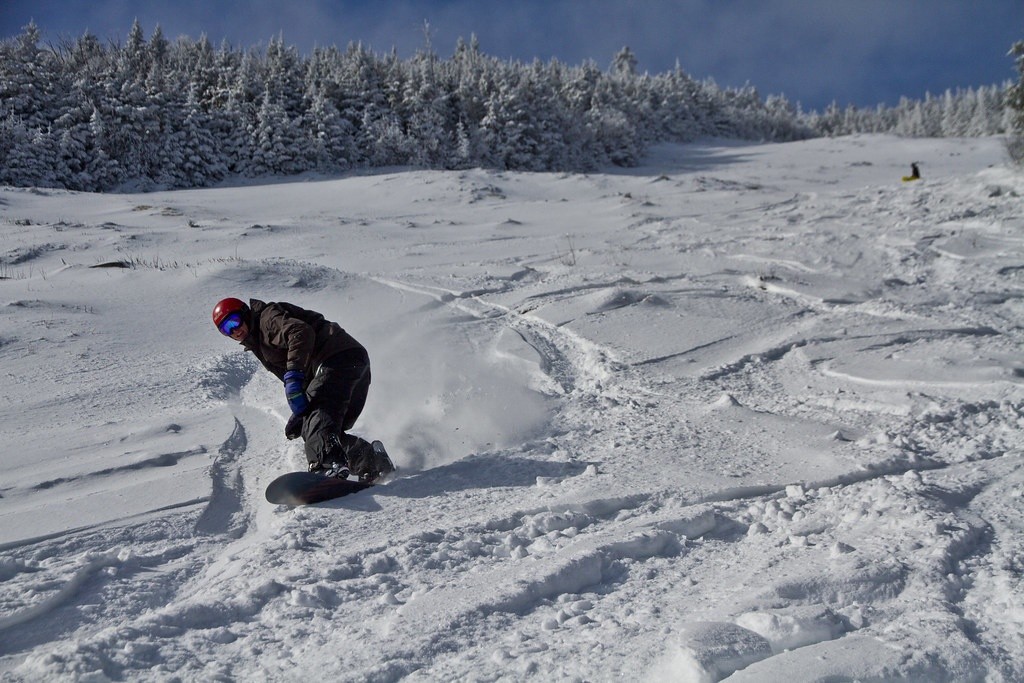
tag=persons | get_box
[902,162,920,181]
[212,297,395,483]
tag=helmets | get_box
[212,298,249,336]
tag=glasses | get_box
[219,311,244,335]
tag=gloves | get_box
[283,372,310,417]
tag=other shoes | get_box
[315,462,349,479]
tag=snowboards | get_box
[264,469,374,507]
